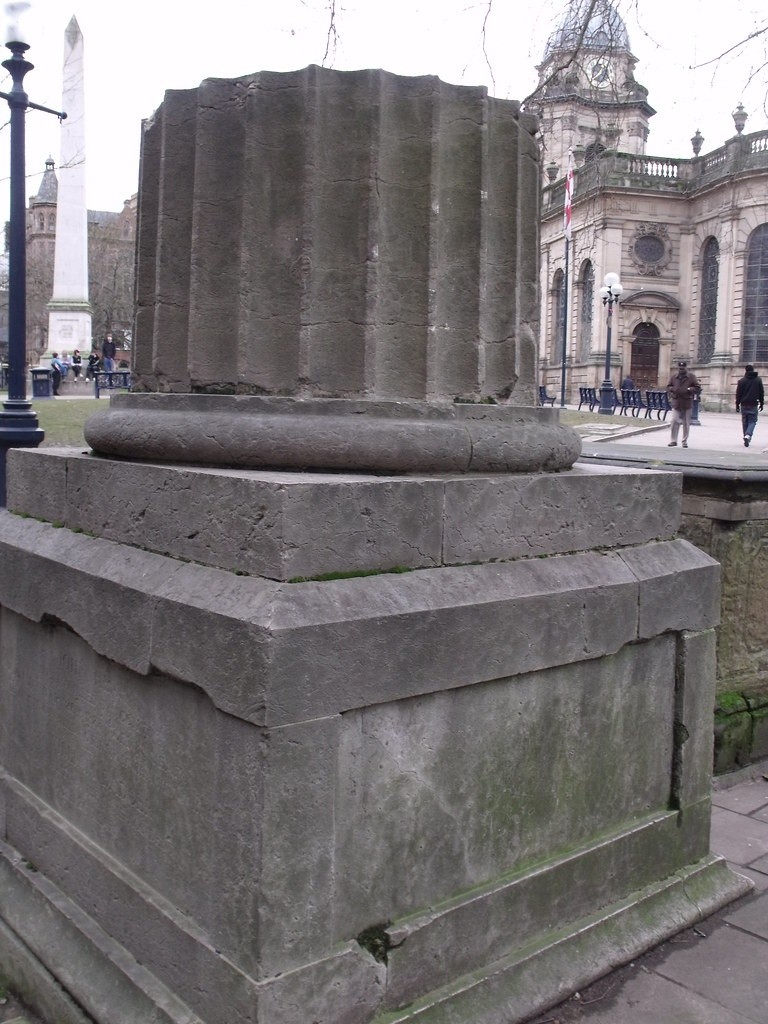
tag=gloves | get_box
[736,406,740,412]
[758,405,763,412]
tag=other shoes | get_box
[80,375,83,377]
[86,378,89,383]
[93,378,95,381]
[53,392,60,395]
[683,444,688,448]
[74,379,77,381]
[668,442,677,446]
[743,435,750,447]
[62,377,66,383]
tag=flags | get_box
[564,161,575,237]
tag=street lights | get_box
[596,272,622,414]
[0,2,67,505]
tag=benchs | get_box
[539,386,556,407]
[94,371,131,398]
[620,389,651,419]
[611,389,622,414]
[645,390,672,421]
[578,388,600,412]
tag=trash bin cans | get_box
[29,369,55,396]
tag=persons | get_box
[61,351,70,379]
[102,333,116,381]
[621,375,634,405]
[737,365,764,447]
[71,349,83,382]
[668,362,700,449]
[51,352,63,396]
[86,352,100,383]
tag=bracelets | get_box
[688,388,690,391]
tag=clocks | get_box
[586,56,615,88]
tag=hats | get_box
[678,362,687,368]
